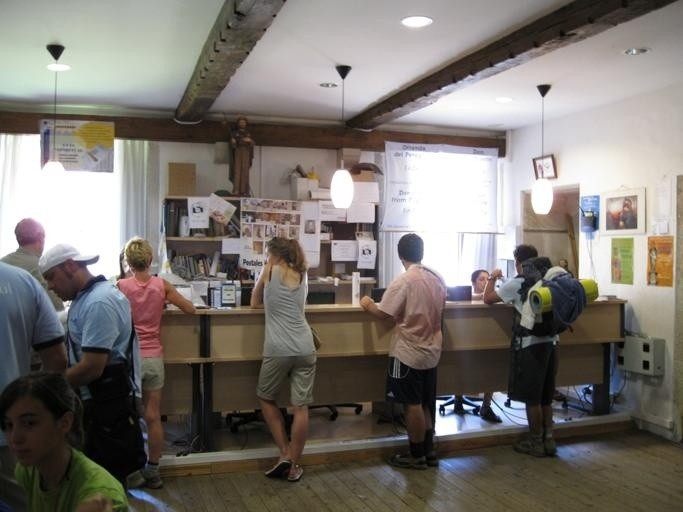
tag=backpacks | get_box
[513,256,569,338]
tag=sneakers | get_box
[146,471,163,489]
[514,437,544,457]
[542,437,558,456]
[425,450,438,468]
[387,451,428,472]
[125,471,144,488]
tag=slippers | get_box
[265,460,291,478]
[287,464,304,482]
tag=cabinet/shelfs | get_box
[159,195,380,303]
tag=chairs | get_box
[305,291,363,422]
[436,286,484,416]
[505,388,568,409]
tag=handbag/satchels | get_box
[66,321,148,481]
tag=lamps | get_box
[37,44,71,214]
[531,84,553,215]
[330,65,354,209]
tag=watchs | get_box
[487,274,496,281]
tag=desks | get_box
[157,306,206,445]
[201,300,443,446]
[433,296,628,433]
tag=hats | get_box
[13,217,44,246]
[39,241,100,273]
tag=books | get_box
[163,202,240,237]
[165,253,239,279]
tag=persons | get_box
[250,236,318,482]
[1,371,133,511]
[0,218,67,318]
[1,257,71,512]
[108,250,133,291]
[229,117,255,196]
[37,243,142,495]
[361,233,447,470]
[484,244,558,457]
[115,236,197,488]
[460,270,503,424]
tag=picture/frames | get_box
[532,154,557,181]
[599,187,646,236]
